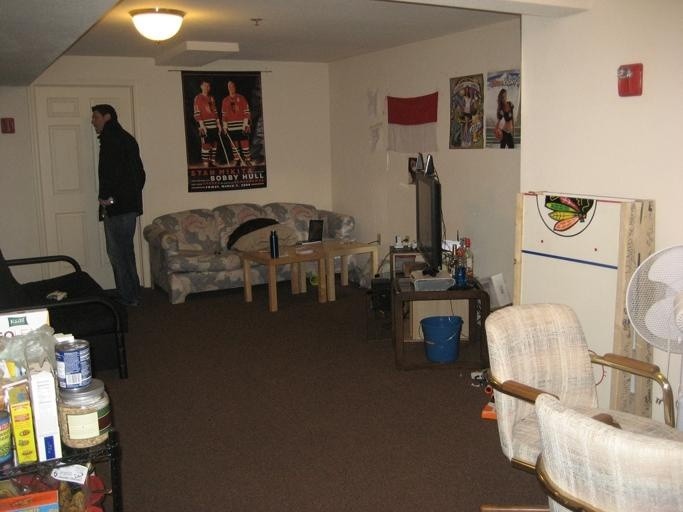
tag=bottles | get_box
[270,229,280,259]
[58,378,112,449]
[450,236,474,288]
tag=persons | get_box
[459,85,476,147]
[495,89,514,149]
[194,78,220,168]
[221,80,255,167]
[92,104,145,307]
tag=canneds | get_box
[54,339,92,392]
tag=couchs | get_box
[143,202,356,305]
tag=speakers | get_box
[423,153,436,177]
[414,151,424,171]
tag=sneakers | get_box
[113,295,143,306]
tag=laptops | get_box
[297,219,323,245]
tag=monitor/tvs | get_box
[414,172,442,277]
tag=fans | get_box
[624,244,683,429]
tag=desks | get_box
[239,246,326,312]
[301,241,379,304]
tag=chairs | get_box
[481,301,675,512]
[1,246,131,382]
[532,394,683,512]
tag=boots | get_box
[208,160,216,167]
[234,159,252,166]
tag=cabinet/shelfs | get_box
[0,428,124,512]
[392,269,491,370]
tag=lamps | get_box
[127,7,186,42]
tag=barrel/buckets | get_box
[419,315,464,364]
